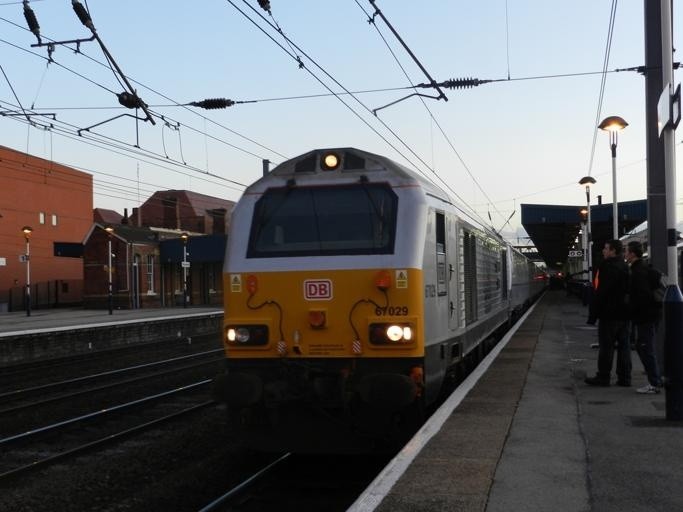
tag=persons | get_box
[589,248,619,352]
[548,270,594,307]
[625,240,664,394]
[583,238,633,387]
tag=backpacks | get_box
[648,267,669,312]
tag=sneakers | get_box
[590,341,600,349]
[584,376,662,394]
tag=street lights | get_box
[180,233,189,308]
[598,117,629,240]
[579,177,596,307]
[104,225,114,315]
[21,226,33,317]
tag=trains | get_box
[223,148,546,407]
